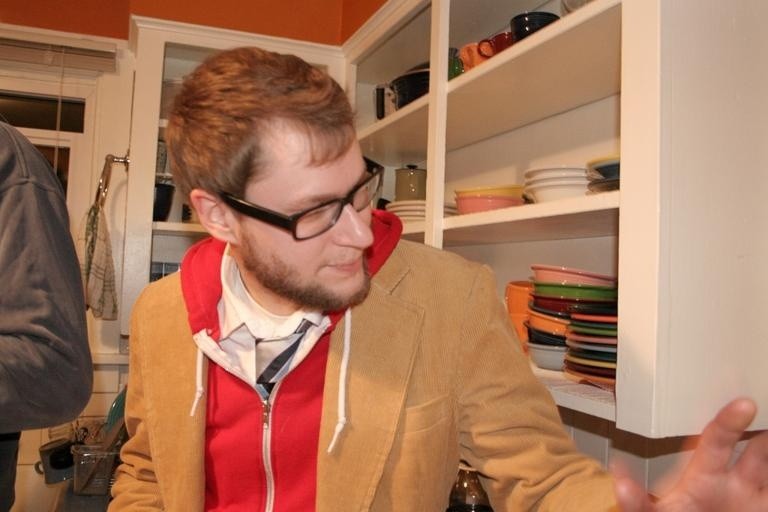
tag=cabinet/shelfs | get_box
[121,13,344,338]
[342,0,767,438]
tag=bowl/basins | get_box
[503,280,533,354]
[523,263,616,372]
[521,166,591,204]
[389,60,430,110]
[455,185,526,216]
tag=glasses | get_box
[208,155,384,242]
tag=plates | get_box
[107,385,126,428]
[563,311,617,394]
[386,199,457,227]
[589,161,620,191]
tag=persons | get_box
[107,45,768,512]
[0,122,93,512]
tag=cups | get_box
[460,31,513,73]
[48,423,77,447]
[155,181,175,222]
[508,11,560,43]
[34,437,75,486]
